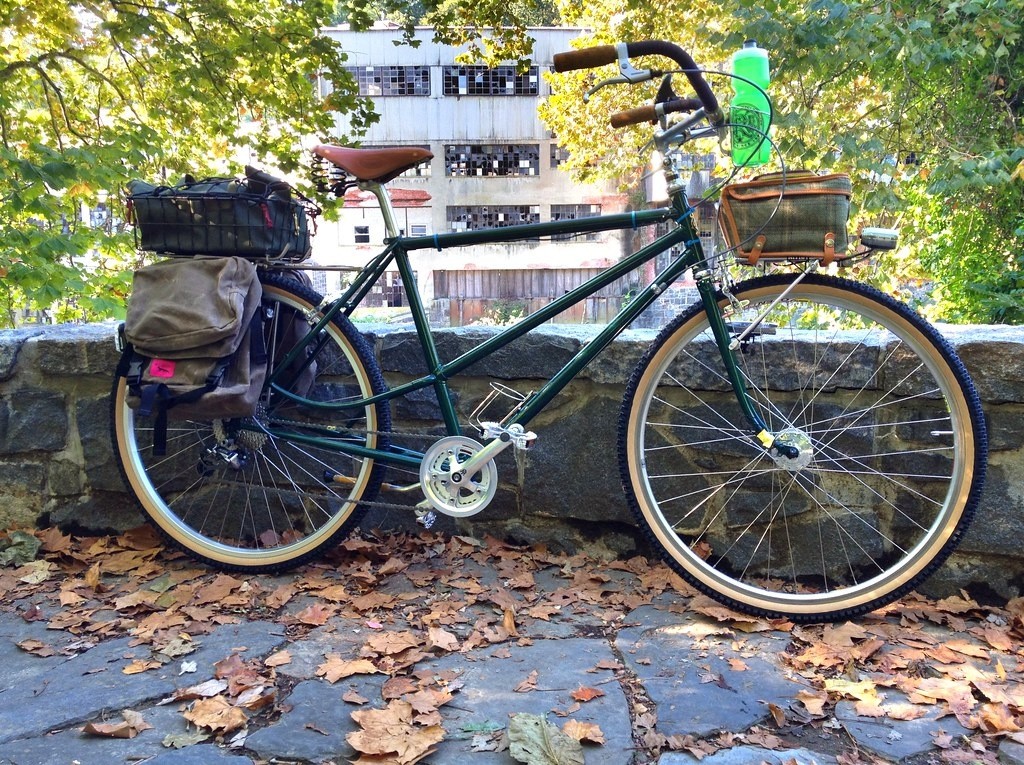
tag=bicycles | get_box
[108,40,988,624]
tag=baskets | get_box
[126,196,310,261]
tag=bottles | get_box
[731,39,770,167]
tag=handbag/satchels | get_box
[123,255,263,358]
[125,321,267,419]
[718,169,851,265]
[264,267,318,408]
